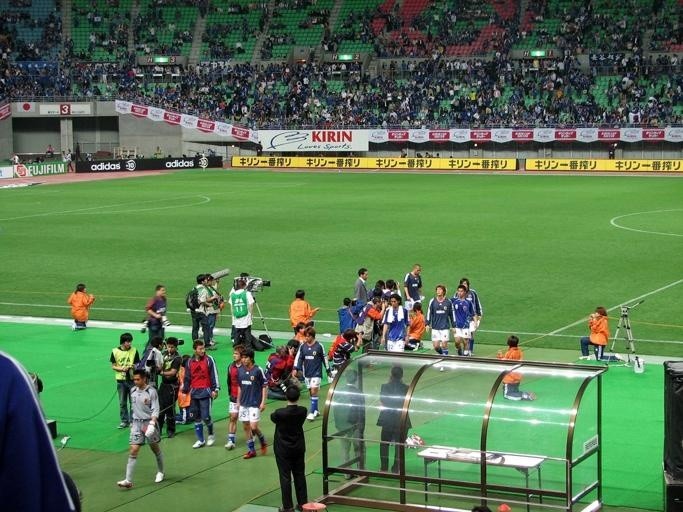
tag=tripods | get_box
[606,306,641,368]
[252,293,269,334]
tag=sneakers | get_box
[210,341,215,346]
[225,441,235,448]
[344,473,354,479]
[579,356,590,360]
[413,343,419,351]
[419,343,423,349]
[314,411,320,416]
[193,439,205,448]
[296,504,303,511]
[205,346,217,350]
[71,320,75,331]
[207,435,214,445]
[528,391,536,400]
[614,353,625,362]
[168,431,174,438]
[440,366,446,371]
[243,450,256,459]
[279,507,295,512]
[117,422,129,429]
[261,441,268,454]
[155,472,165,482]
[117,480,132,488]
[306,413,314,421]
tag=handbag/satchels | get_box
[240,332,265,351]
[259,335,272,347]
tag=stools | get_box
[301,502,329,512]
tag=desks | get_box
[417,445,547,512]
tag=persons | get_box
[1,1,682,172]
[68,283,95,330]
[497,336,536,401]
[0,355,81,512]
[108,264,482,509]
[578,307,623,361]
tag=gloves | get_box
[145,420,156,437]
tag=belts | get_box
[134,419,151,422]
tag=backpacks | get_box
[186,287,200,309]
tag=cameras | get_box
[354,330,364,341]
[208,268,229,281]
[246,277,270,292]
[164,338,184,346]
